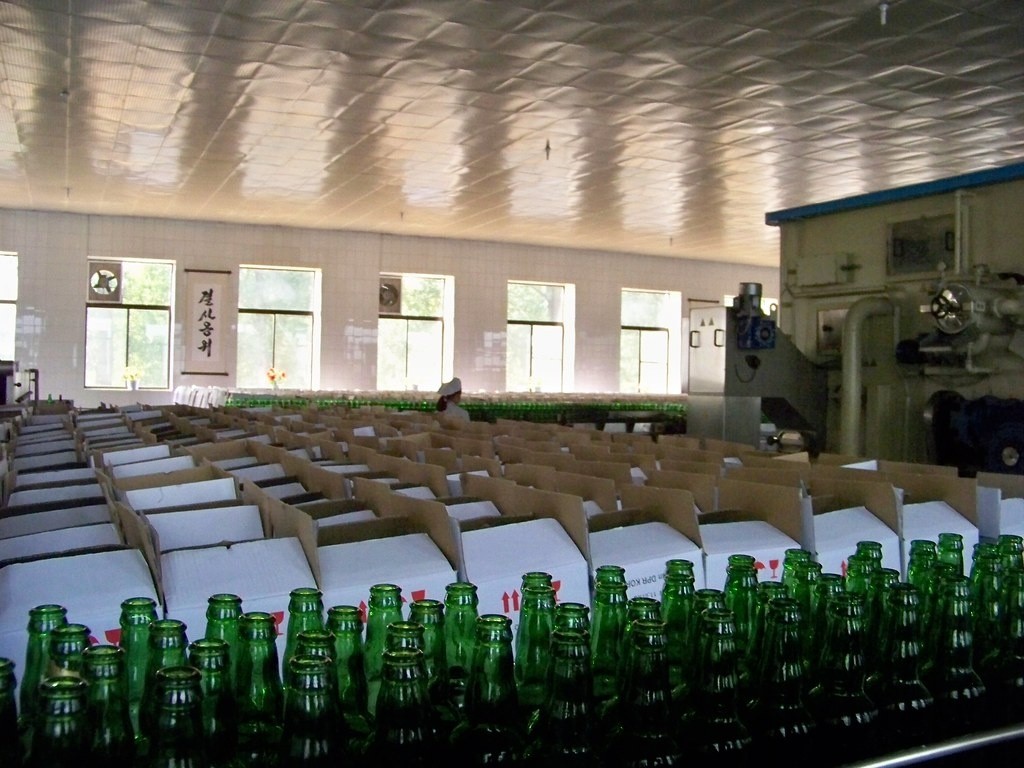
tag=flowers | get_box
[122,366,141,379]
[266,368,285,383]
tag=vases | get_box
[274,381,279,389]
[129,379,139,389]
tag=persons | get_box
[436,377,470,423]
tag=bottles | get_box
[222,389,690,416]
[47,394,52,407]
[0,528,1024,766]
[100,402,113,409]
[59,394,63,402]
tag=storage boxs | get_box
[0,399,1024,717]
